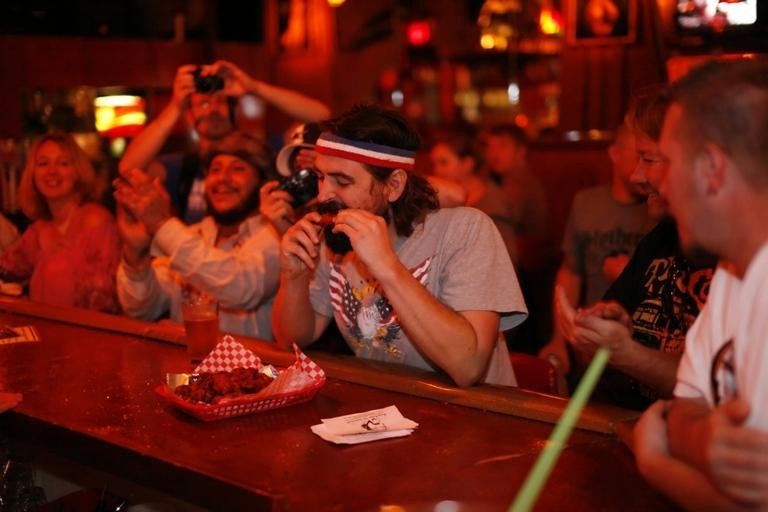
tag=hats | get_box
[208,132,274,181]
[276,122,320,177]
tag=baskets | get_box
[154,367,327,422]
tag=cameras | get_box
[278,167,318,208]
[189,63,224,93]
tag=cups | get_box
[181,284,221,362]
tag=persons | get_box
[630,59,767,512]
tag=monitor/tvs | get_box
[675,0,768,37]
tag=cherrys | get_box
[174,367,274,403]
